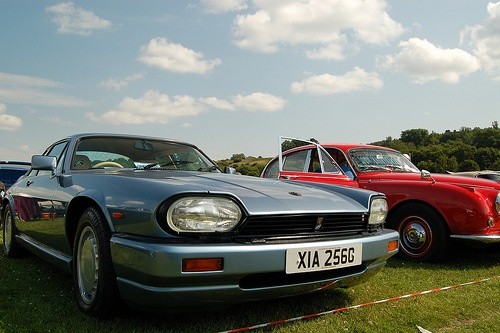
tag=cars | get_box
[1,132,398,317]
[261,136,499,261]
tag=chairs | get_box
[71,155,91,170]
